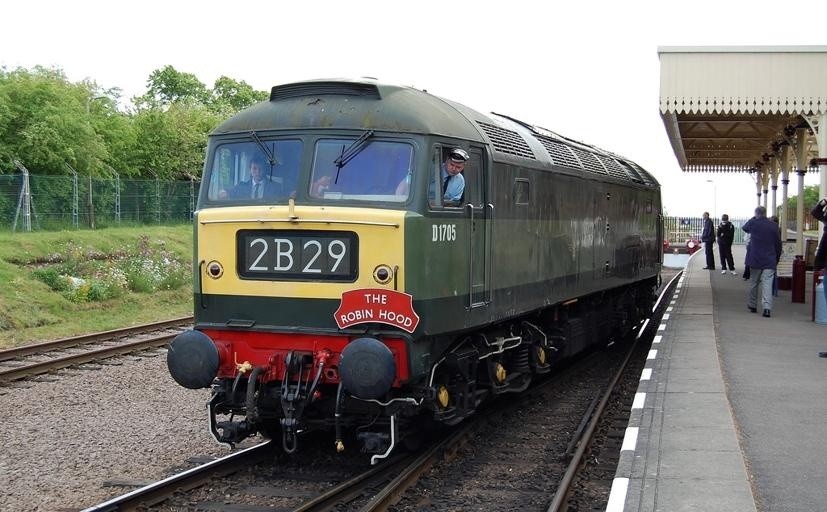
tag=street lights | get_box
[85,90,116,232]
[705,178,716,243]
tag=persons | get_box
[811,198,827,358]
[717,214,737,275]
[742,206,782,318]
[743,232,751,281]
[701,212,716,269]
[219,158,283,200]
[395,147,469,201]
[770,215,779,296]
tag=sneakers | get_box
[762,309,771,317]
[720,270,726,274]
[750,309,757,313]
[730,270,737,275]
[702,265,715,270]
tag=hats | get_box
[448,148,470,163]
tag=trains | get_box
[164,74,664,464]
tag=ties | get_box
[253,183,259,199]
[443,176,450,194]
